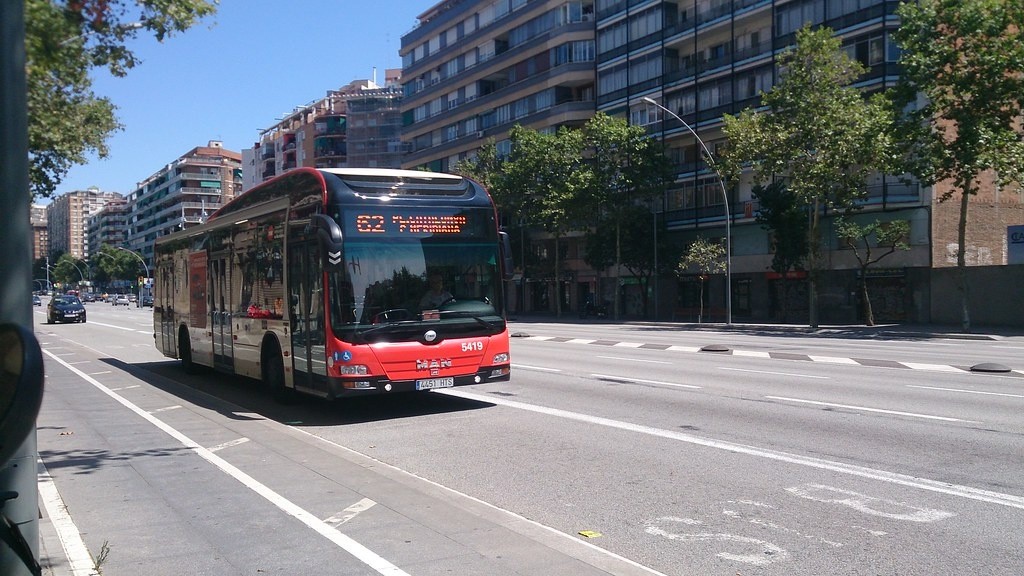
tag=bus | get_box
[154,167,510,406]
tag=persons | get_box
[419,275,453,309]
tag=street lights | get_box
[63,259,84,281]
[637,95,731,324]
[98,252,115,263]
[118,246,151,306]
[41,266,57,282]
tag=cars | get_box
[135,295,154,308]
[126,293,137,302]
[33,289,64,297]
[32,295,41,306]
[112,295,130,307]
[66,290,117,303]
[47,295,86,324]
